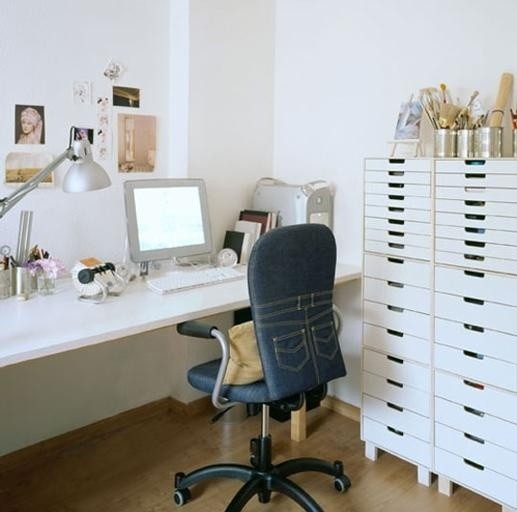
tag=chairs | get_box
[173,222,351,511]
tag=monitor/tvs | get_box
[124,178,213,273]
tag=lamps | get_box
[0,140,112,218]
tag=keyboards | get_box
[146,265,246,294]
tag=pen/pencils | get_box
[0,244,49,270]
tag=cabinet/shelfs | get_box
[361,157,517,512]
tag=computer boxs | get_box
[251,177,333,229]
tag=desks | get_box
[0,248,363,370]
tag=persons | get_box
[75,128,89,145]
[16,106,43,144]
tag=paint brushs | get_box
[416,84,488,130]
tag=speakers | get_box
[218,248,238,268]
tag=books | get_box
[221,207,278,265]
